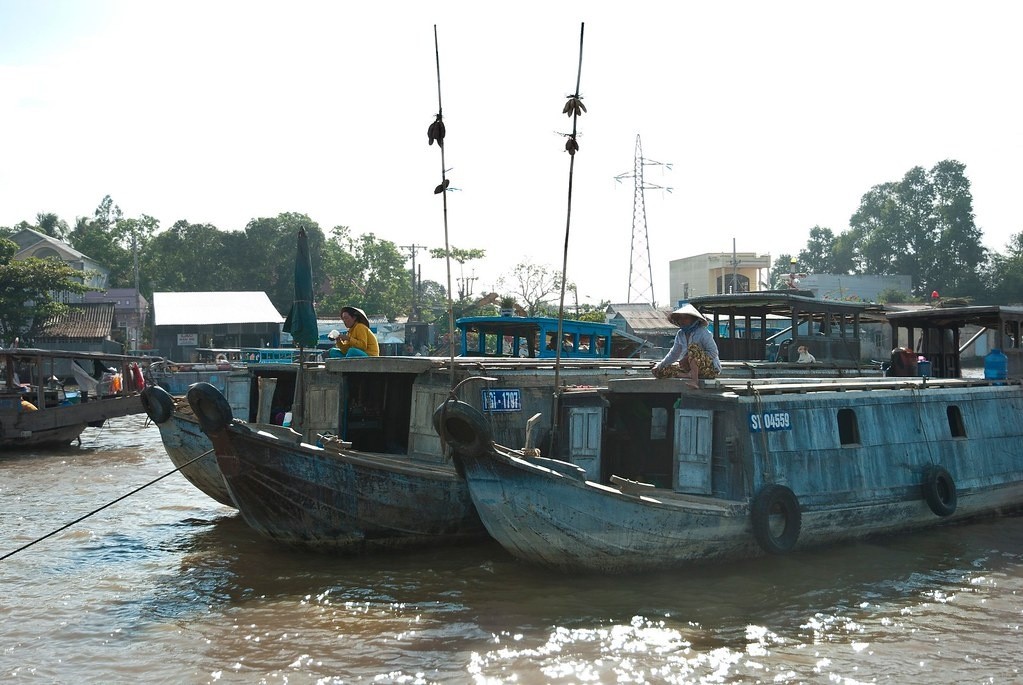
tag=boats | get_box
[168,348,248,371]
[433,21,1023,571]
[227,347,325,377]
[139,224,613,510]
[0,347,176,450]
[185,24,930,551]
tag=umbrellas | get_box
[282,226,318,421]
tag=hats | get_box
[48,375,59,383]
[341,306,369,328]
[668,304,708,328]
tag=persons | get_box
[420,343,429,355]
[769,341,778,361]
[406,344,414,355]
[652,305,722,390]
[329,306,379,358]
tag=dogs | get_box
[796,345,823,364]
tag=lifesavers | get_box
[750,484,802,555]
[140,386,175,423]
[186,382,233,431]
[432,399,492,457]
[216,353,227,364]
[923,465,957,517]
